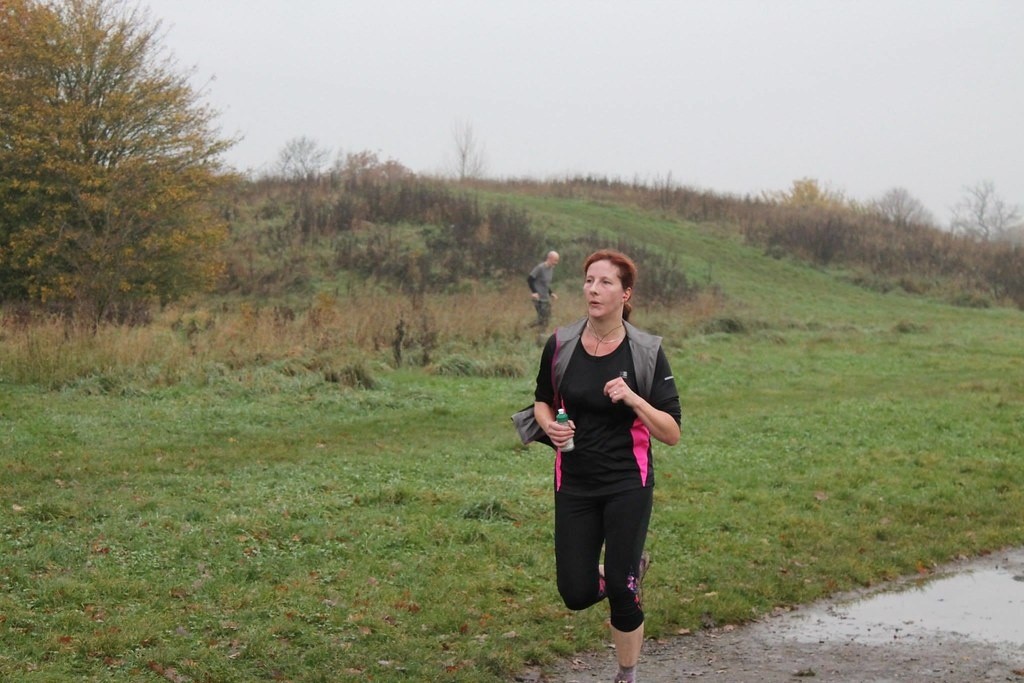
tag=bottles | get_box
[556,408,575,452]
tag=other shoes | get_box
[638,550,651,582]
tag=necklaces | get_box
[587,324,622,344]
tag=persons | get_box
[527,250,560,334]
[535,250,682,683]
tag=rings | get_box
[613,391,618,395]
